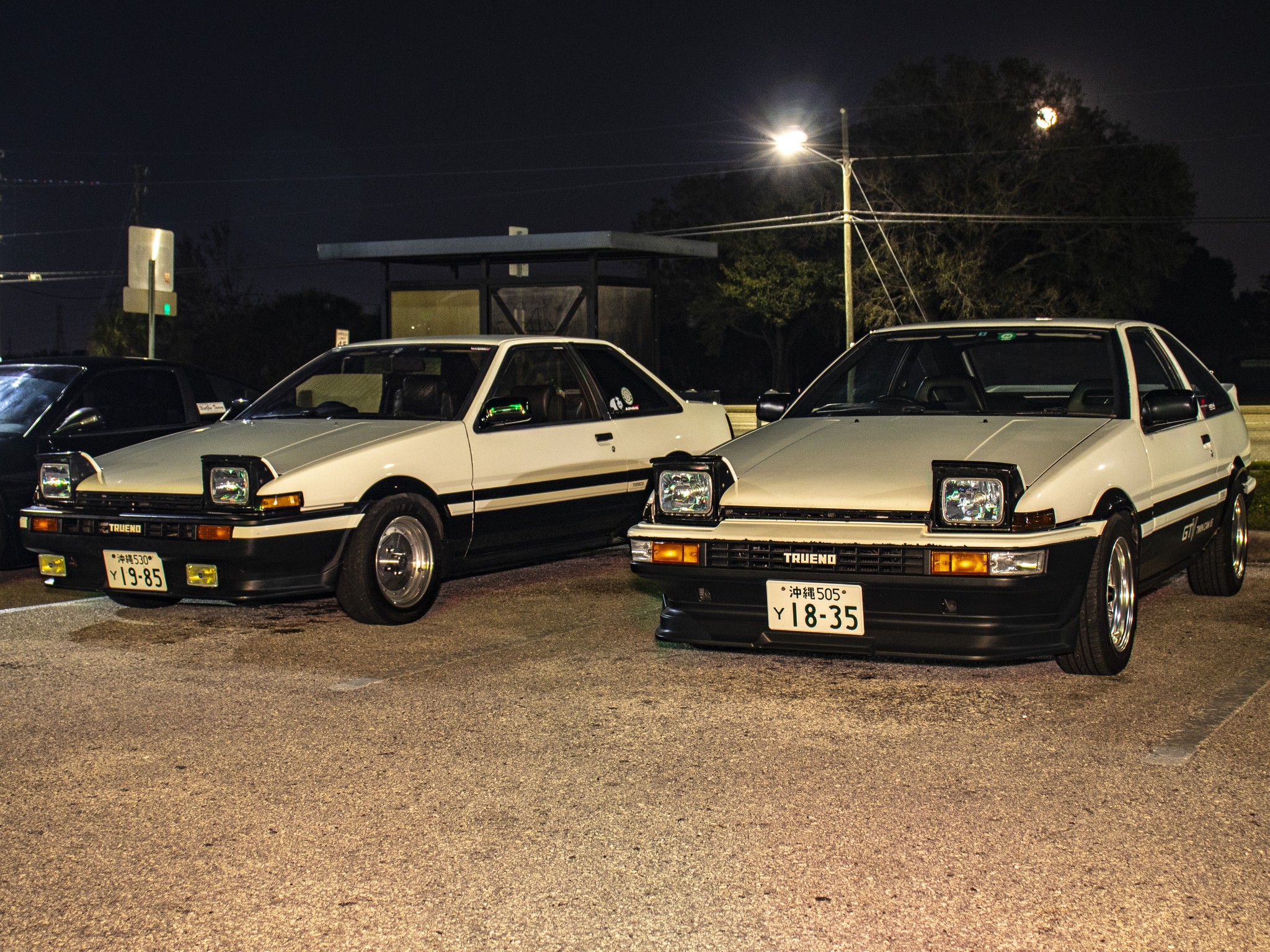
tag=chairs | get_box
[392,374,454,421]
[918,374,987,411]
[499,382,565,422]
[1063,377,1114,413]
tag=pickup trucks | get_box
[0,355,306,572]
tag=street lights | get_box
[774,108,861,406]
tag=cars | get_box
[627,318,1258,676]
[18,338,729,627]
